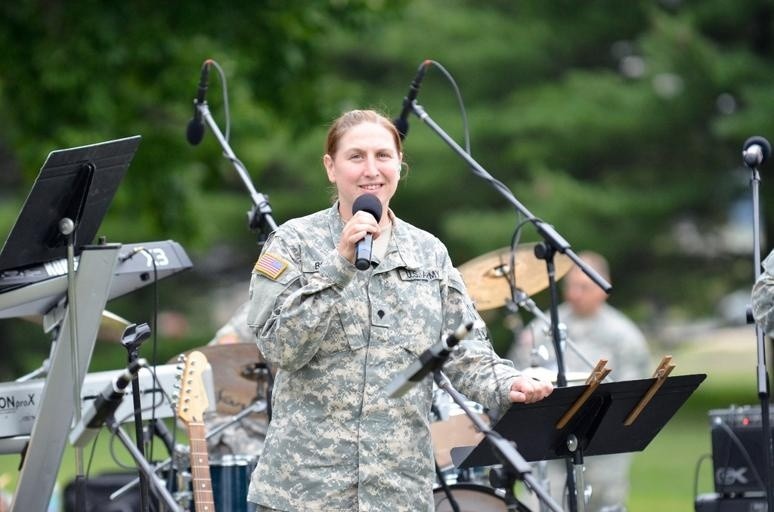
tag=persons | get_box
[502,251,655,512]
[247,109,554,512]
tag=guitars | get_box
[172,350,215,512]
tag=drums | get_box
[182,453,258,512]
[433,465,542,512]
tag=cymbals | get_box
[456,243,573,312]
[167,344,278,420]
[431,414,490,471]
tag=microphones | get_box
[386,326,467,399]
[393,66,422,142]
[741,135,771,168]
[352,194,382,270]
[69,359,141,448]
[187,67,209,145]
